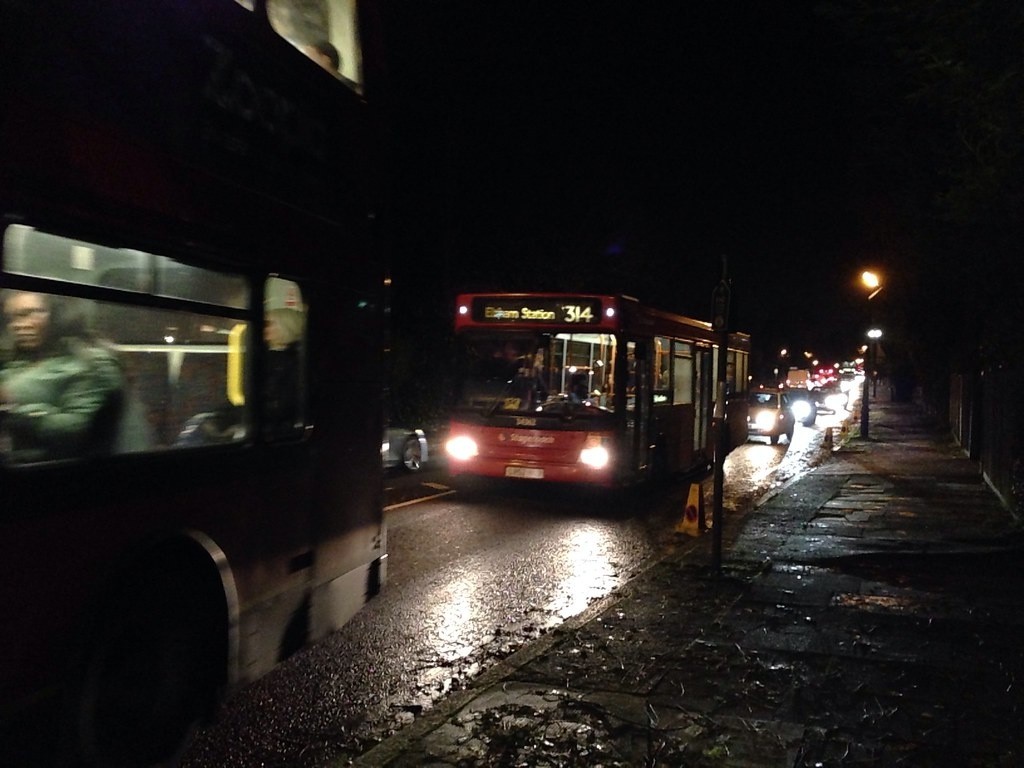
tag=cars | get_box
[785,388,820,429]
[806,367,859,412]
[378,414,431,474]
[750,388,795,445]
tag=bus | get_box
[442,288,751,494]
[0,215,399,768]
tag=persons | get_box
[1,287,129,465]
[264,321,287,353]
[726,376,735,396]
[658,364,670,388]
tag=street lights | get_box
[858,270,882,438]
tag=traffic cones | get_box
[672,483,709,539]
